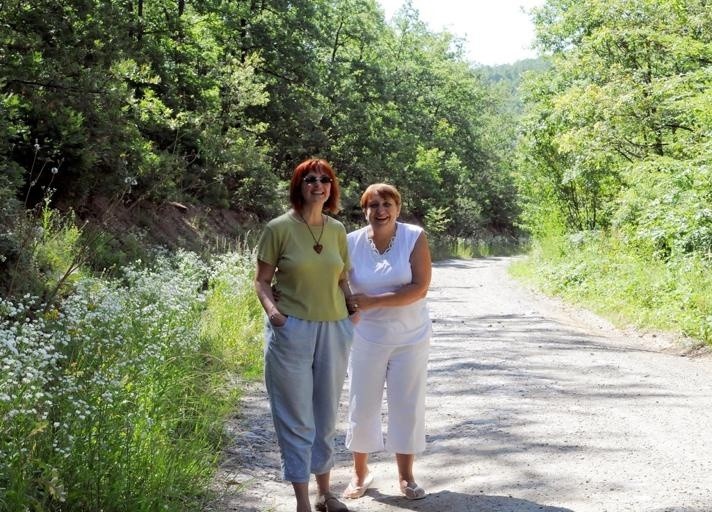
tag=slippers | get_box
[399,480,425,500]
[343,476,374,499]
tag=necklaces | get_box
[299,212,324,254]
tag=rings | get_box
[355,304,357,307]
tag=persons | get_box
[345,185,432,500]
[257,159,359,511]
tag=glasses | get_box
[303,175,333,183]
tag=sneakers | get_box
[315,496,347,512]
